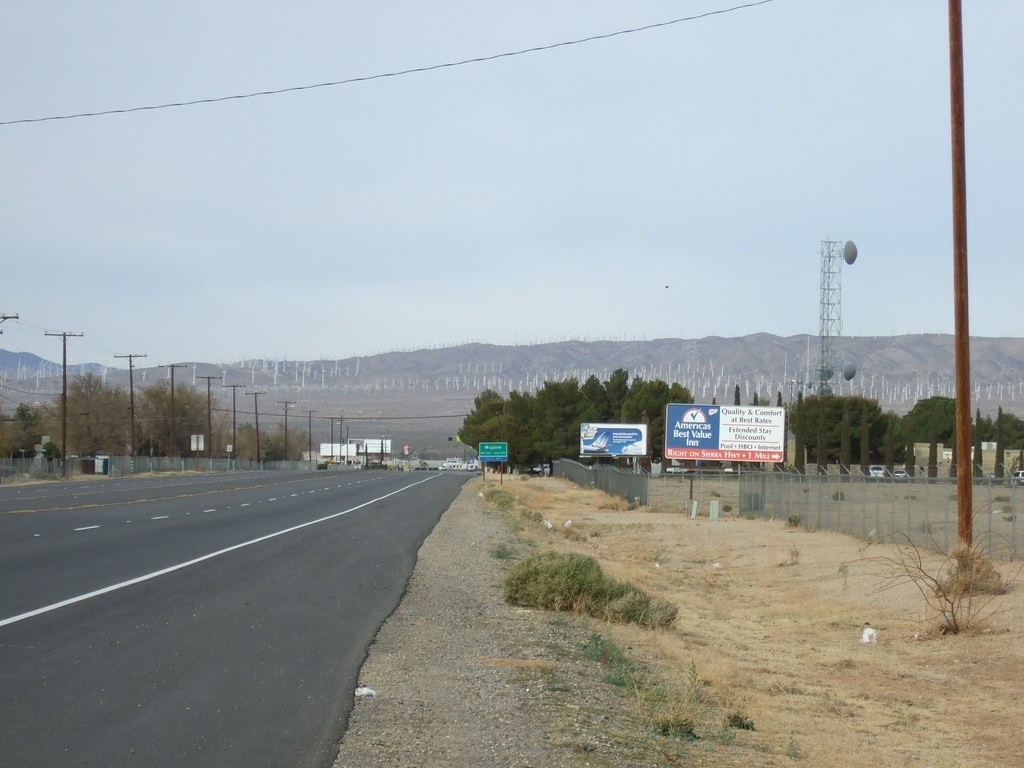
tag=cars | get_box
[892,470,906,478]
[1013,471,1024,483]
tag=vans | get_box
[869,464,885,478]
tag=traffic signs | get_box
[665,403,786,463]
